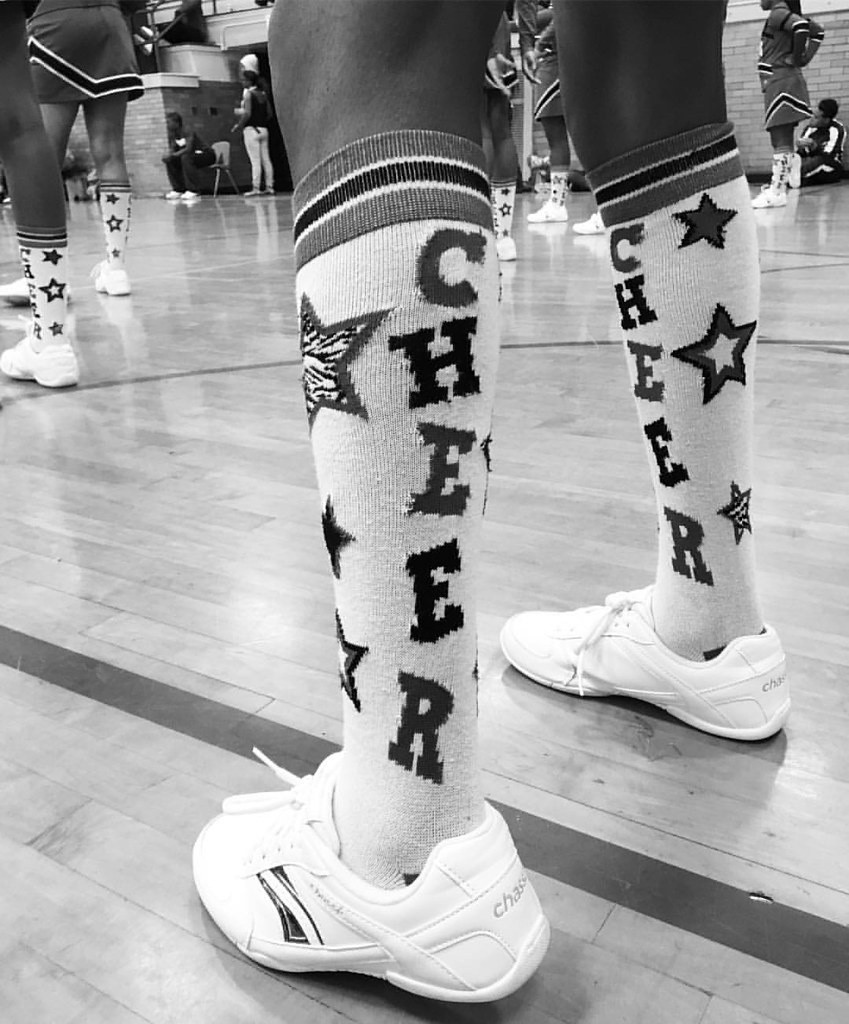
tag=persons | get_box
[192,0,791,1003]
[0,0,146,306]
[751,0,824,209]
[134,0,207,55]
[231,54,275,196]
[161,112,216,199]
[760,99,847,190]
[0,0,79,388]
[483,0,605,261]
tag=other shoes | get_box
[133,25,160,55]
[164,190,200,199]
[244,186,274,196]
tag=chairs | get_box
[208,141,240,199]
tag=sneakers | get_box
[496,235,516,260]
[0,321,80,389]
[527,199,568,223]
[753,188,787,208]
[497,579,795,742]
[95,269,132,296]
[0,277,31,306]
[572,212,606,235]
[789,153,801,188]
[188,749,553,1004]
